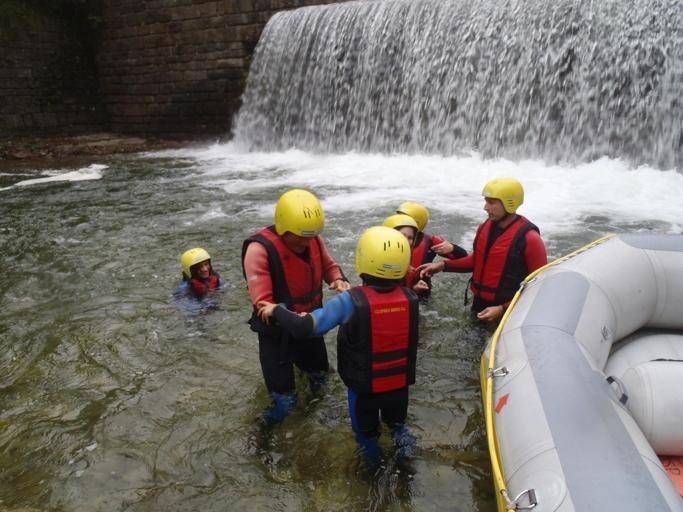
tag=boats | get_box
[478,231,681,511]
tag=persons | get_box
[414,177,549,332]
[171,248,222,311]
[241,189,350,425]
[251,227,419,456]
[395,201,467,294]
[382,214,429,293]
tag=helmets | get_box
[482,176,525,214]
[383,202,429,247]
[274,189,324,238]
[356,225,411,283]
[181,247,211,279]
